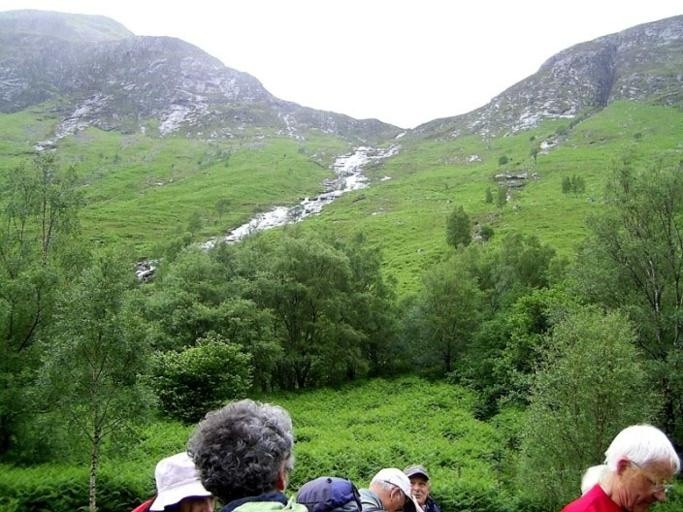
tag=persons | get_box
[295,468,411,512]
[144,450,218,512]
[185,399,310,512]
[556,423,680,512]
[401,465,439,512]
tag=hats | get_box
[149,453,213,511]
[297,464,429,512]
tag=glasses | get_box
[631,462,673,492]
